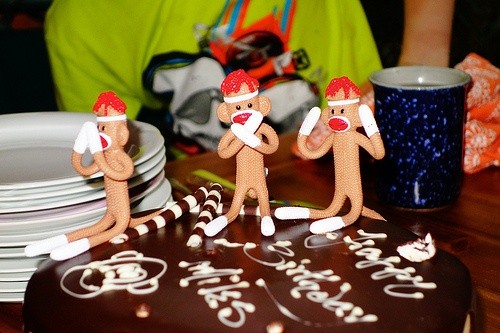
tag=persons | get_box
[45,0,381,162]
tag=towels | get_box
[453,52,500,174]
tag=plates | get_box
[0,112,173,303]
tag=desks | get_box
[0,130,500,333]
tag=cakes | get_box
[23,69,476,333]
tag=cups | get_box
[368,65,471,212]
